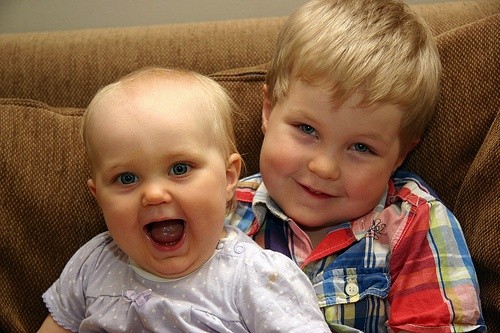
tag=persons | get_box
[224,1,487,333]
[37,66,330,333]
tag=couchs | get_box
[0,0,500,333]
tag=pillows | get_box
[0,14,500,333]
